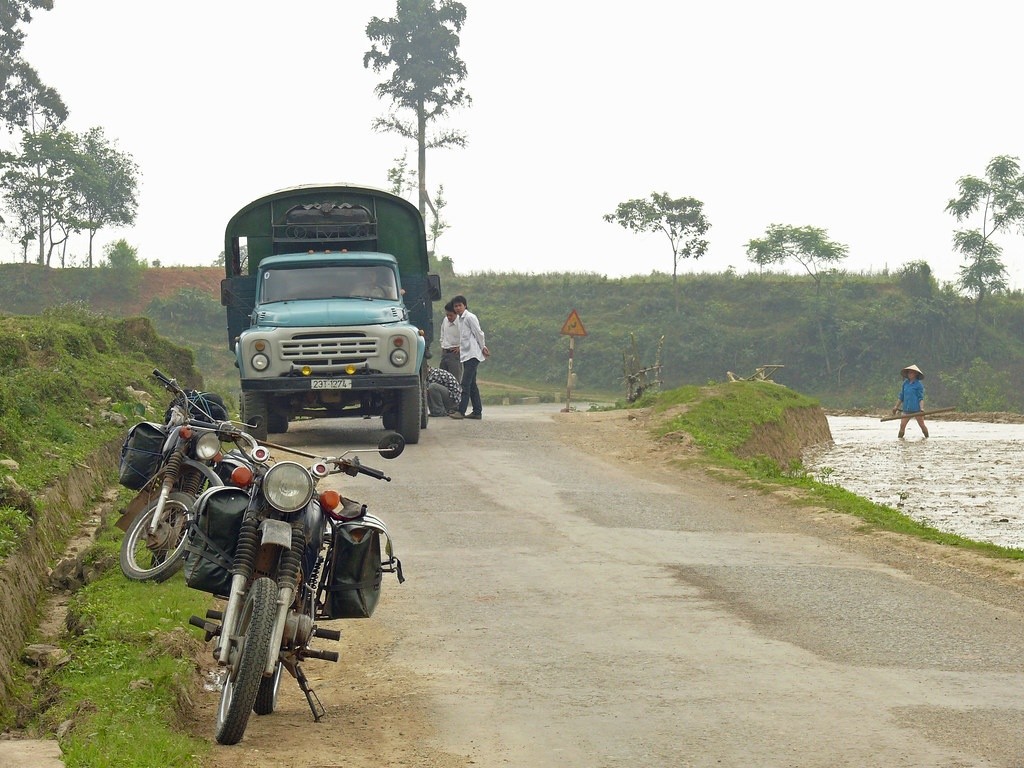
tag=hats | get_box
[901,364,923,379]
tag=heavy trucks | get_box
[219,180,443,444]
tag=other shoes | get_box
[428,412,447,417]
[449,411,464,419]
[464,412,481,419]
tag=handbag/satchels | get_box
[322,514,405,618]
[119,421,169,492]
[181,486,251,597]
[163,388,227,427]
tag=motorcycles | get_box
[184,412,407,745]
[116,367,265,582]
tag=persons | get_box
[361,399,384,421]
[426,364,463,417]
[892,364,929,438]
[439,302,464,384]
[448,295,490,419]
